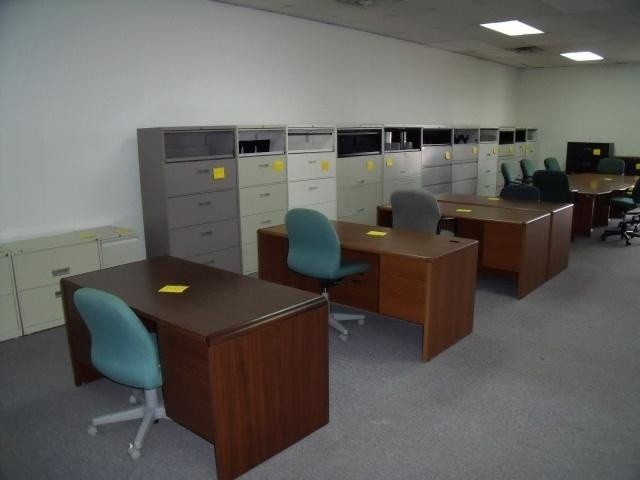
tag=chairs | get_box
[280,206,371,340]
[390,189,455,236]
[499,156,640,245]
[70,286,177,461]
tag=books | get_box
[384,130,393,144]
[398,130,408,149]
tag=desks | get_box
[438,192,573,281]
[56,252,329,479]
[257,218,479,362]
[376,200,551,301]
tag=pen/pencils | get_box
[449,241,460,242]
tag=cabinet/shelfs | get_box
[384,128,423,204]
[479,128,499,193]
[284,129,337,223]
[452,127,479,193]
[137,128,242,273]
[235,128,288,273]
[422,126,452,197]
[499,130,515,189]
[0,225,140,342]
[526,127,541,175]
[514,128,527,181]
[336,129,384,224]
